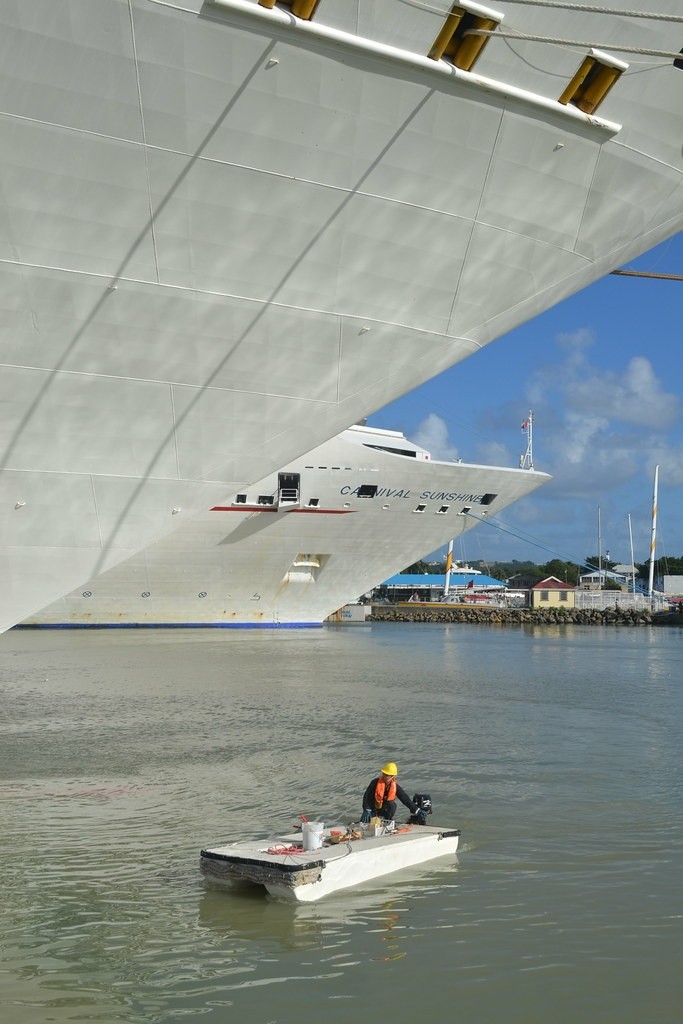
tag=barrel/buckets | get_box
[301,822,325,851]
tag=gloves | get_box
[415,808,428,818]
[360,809,373,823]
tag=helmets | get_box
[380,762,398,776]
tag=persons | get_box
[360,761,427,822]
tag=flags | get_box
[467,580,473,589]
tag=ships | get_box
[9,409,554,629]
[0,0,683,632]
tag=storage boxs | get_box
[360,819,396,836]
